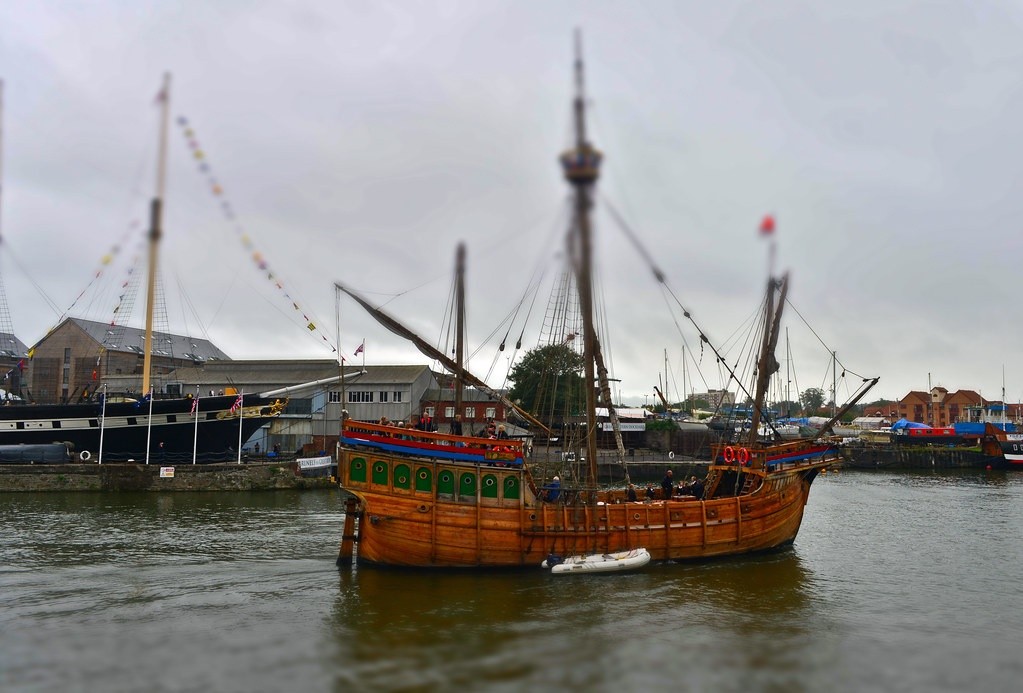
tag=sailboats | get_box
[325,29,878,567]
[0,60,371,459]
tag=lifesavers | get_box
[724,446,734,462]
[738,448,748,464]
[492,445,510,466]
[80,450,91,461]
[669,452,675,459]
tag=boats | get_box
[539,548,651,576]
[705,363,1023,466]
